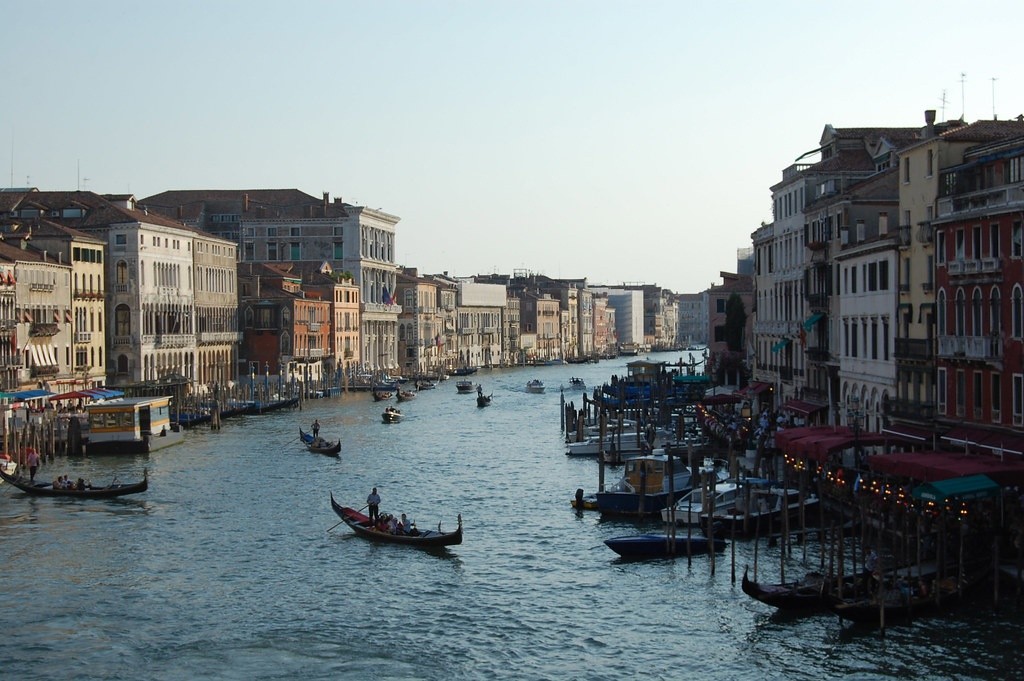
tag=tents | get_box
[865,450,1023,481]
[777,424,904,463]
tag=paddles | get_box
[282,430,311,448]
[3,460,37,495]
[326,501,374,532]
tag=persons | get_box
[367,488,421,536]
[26,449,93,491]
[311,420,334,450]
[863,544,880,596]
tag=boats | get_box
[477,394,493,406]
[561,373,817,537]
[602,532,728,559]
[298,427,341,455]
[456,380,478,393]
[0,463,153,497]
[525,382,545,393]
[741,565,885,615]
[167,387,324,426]
[819,576,982,623]
[358,372,410,387]
[414,380,438,390]
[381,412,401,423]
[372,392,392,401]
[329,491,464,548]
[395,388,417,401]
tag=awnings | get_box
[783,400,824,415]
[739,381,772,397]
[882,424,1024,456]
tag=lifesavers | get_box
[642,445,650,454]
